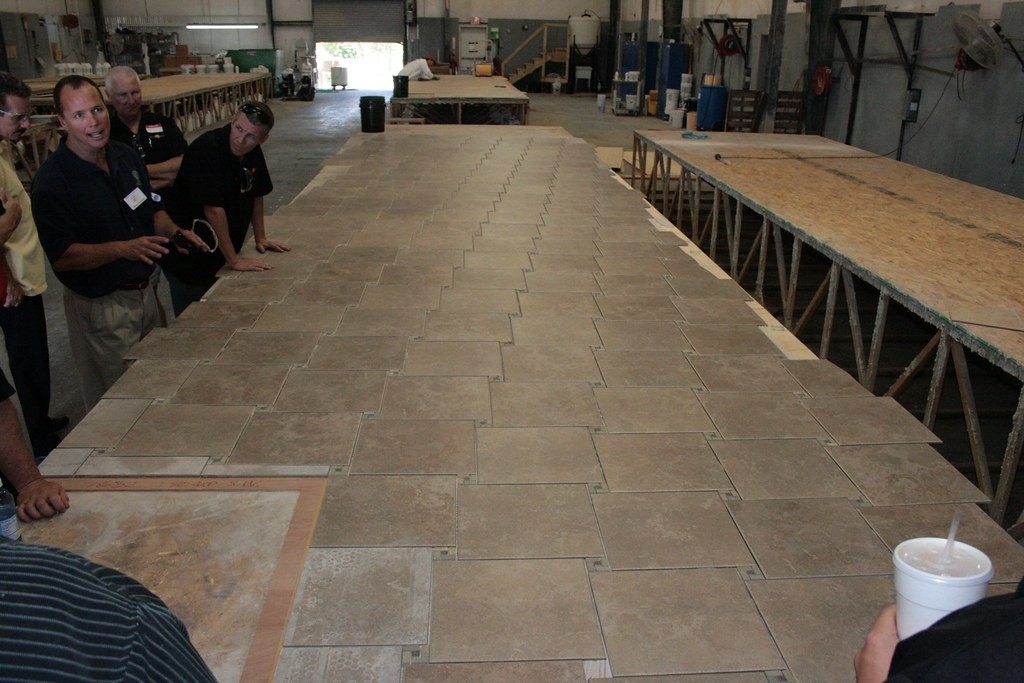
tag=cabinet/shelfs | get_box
[0,10,98,82]
[620,39,689,116]
[459,23,489,74]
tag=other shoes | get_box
[49,416,69,431]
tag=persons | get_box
[164,101,293,307]
[0,535,215,682]
[0,370,67,523]
[399,56,437,80]
[0,73,70,457]
[105,66,187,192]
[856,579,1024,683]
[30,74,209,408]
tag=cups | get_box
[892,537,994,643]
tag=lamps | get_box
[186,23,259,29]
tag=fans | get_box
[950,9,1024,73]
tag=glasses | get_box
[0,107,36,122]
[238,104,268,125]
[239,168,254,193]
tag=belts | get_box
[120,279,149,290]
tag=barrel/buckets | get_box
[359,96,387,133]
[181,57,235,75]
[54,62,111,77]
[393,76,409,98]
[666,73,697,131]
[696,85,726,131]
